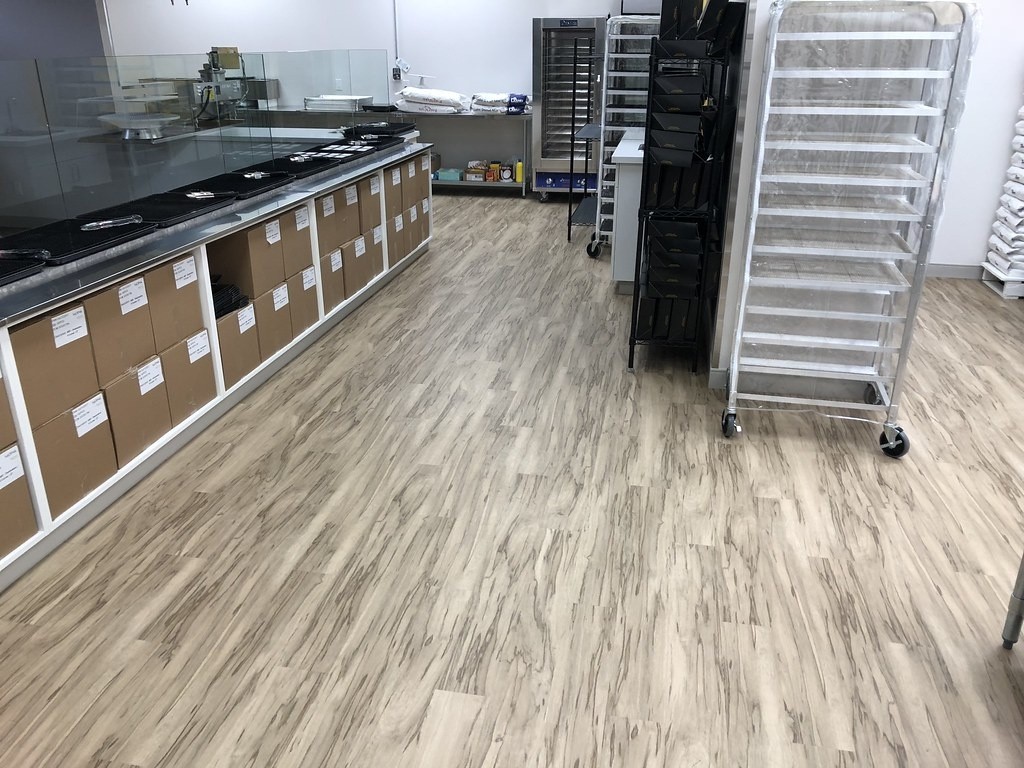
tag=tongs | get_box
[186,192,239,198]
[290,156,329,162]
[356,122,389,128]
[360,135,392,139]
[80,215,142,230]
[348,140,381,146]
[244,170,289,179]
[0,249,50,260]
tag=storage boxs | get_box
[142,252,204,354]
[0,364,17,452]
[33,392,118,522]
[217,151,430,391]
[439,168,463,181]
[9,301,100,431]
[0,441,39,560]
[157,327,216,429]
[99,353,172,470]
[490,161,514,182]
[431,152,441,173]
[463,168,487,181]
[75,274,156,388]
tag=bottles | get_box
[516,159,522,182]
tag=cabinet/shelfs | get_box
[627,38,724,372]
[566,38,650,243]
[236,108,532,199]
[610,138,644,291]
[586,16,662,258]
[721,0,981,455]
